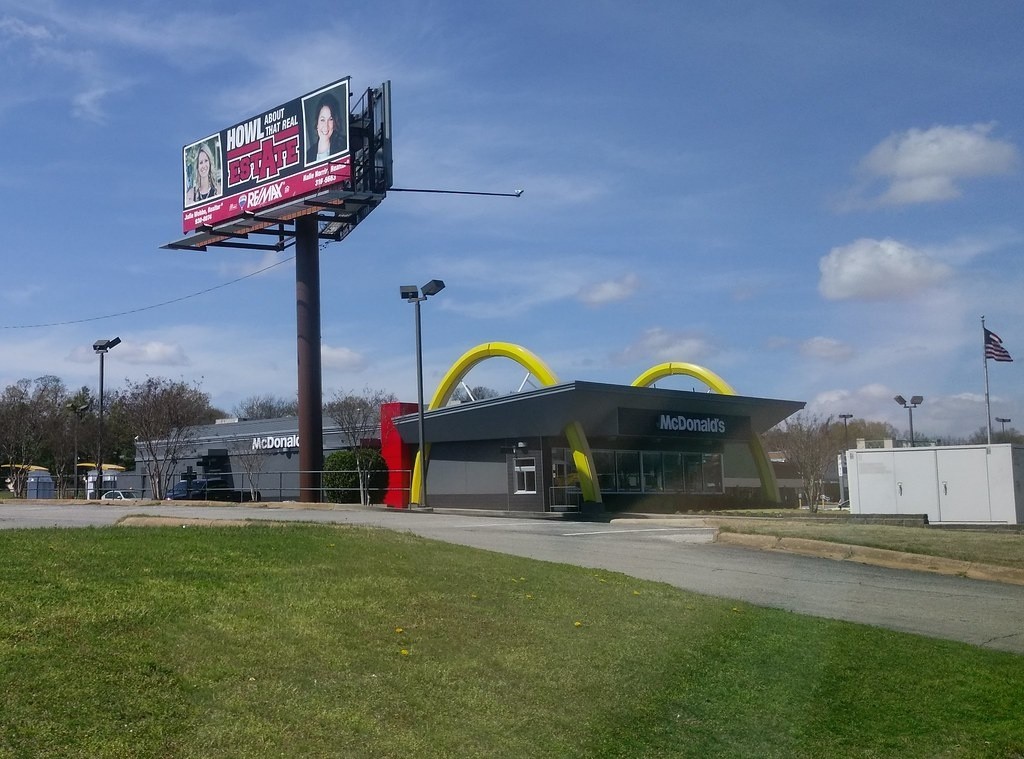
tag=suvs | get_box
[164,479,232,502]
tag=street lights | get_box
[65,403,87,497]
[839,414,854,452]
[400,277,446,512]
[995,417,1011,442]
[894,394,925,448]
[92,337,122,498]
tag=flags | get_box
[980,313,1014,364]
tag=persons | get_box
[836,500,843,508]
[185,146,221,204]
[307,94,347,163]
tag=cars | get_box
[100,490,137,501]
